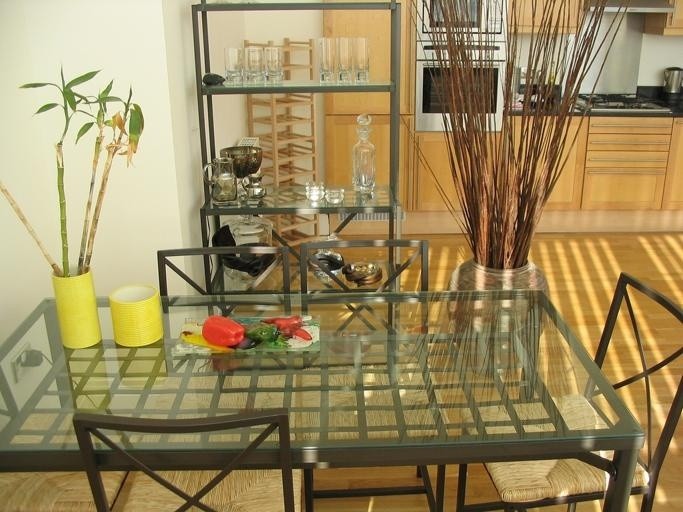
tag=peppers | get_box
[278,327,292,337]
[262,316,302,329]
[294,328,312,341]
[202,316,244,347]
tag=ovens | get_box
[415,41,509,132]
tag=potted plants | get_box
[0,64,146,352]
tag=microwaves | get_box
[415,0,510,42]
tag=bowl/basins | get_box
[219,145,263,178]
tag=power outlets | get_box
[9,342,39,384]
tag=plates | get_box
[175,315,321,355]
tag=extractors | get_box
[587,0,676,14]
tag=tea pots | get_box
[663,67,683,93]
[202,157,238,206]
[240,174,267,199]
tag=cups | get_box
[305,180,324,201]
[334,37,350,84]
[223,47,244,86]
[315,38,335,84]
[349,36,369,84]
[243,48,263,85]
[325,186,344,204]
[264,47,282,85]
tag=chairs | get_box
[139,246,294,449]
[70,405,306,512]
[298,238,449,512]
[456,270,683,512]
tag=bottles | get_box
[351,113,376,193]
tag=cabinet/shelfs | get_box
[412,115,683,213]
[509,0,683,36]
[190,0,404,297]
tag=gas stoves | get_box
[574,93,670,114]
